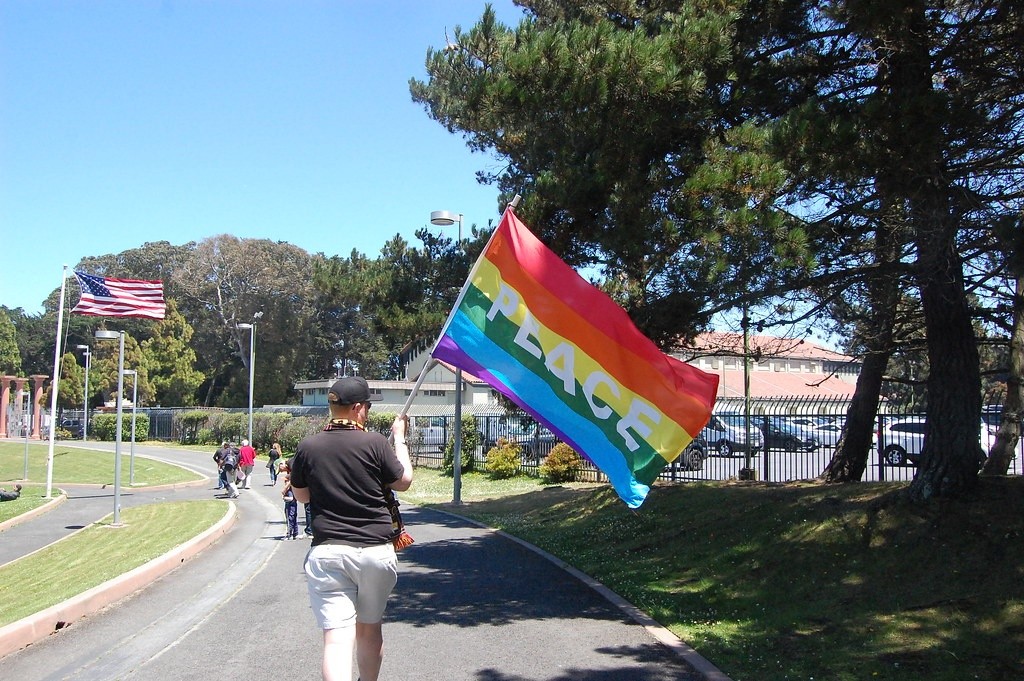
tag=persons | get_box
[213,439,240,498]
[281,459,314,541]
[266,443,282,487]
[239,440,255,488]
[291,377,414,681]
[0,484,22,502]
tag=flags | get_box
[70,270,166,320]
[432,205,719,508]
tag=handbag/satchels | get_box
[266,459,274,468]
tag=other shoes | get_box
[229,493,240,499]
[302,532,313,539]
[281,537,292,542]
[295,533,304,540]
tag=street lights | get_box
[237,322,254,447]
[76,344,92,442]
[430,210,465,506]
[123,368,138,485]
[95,329,125,527]
[20,391,31,480]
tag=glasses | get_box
[352,403,372,409]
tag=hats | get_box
[236,470,246,481]
[328,377,384,405]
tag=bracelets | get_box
[403,441,407,445]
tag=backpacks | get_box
[224,448,237,471]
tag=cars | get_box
[672,405,1018,471]
[508,425,555,462]
[60,416,81,436]
